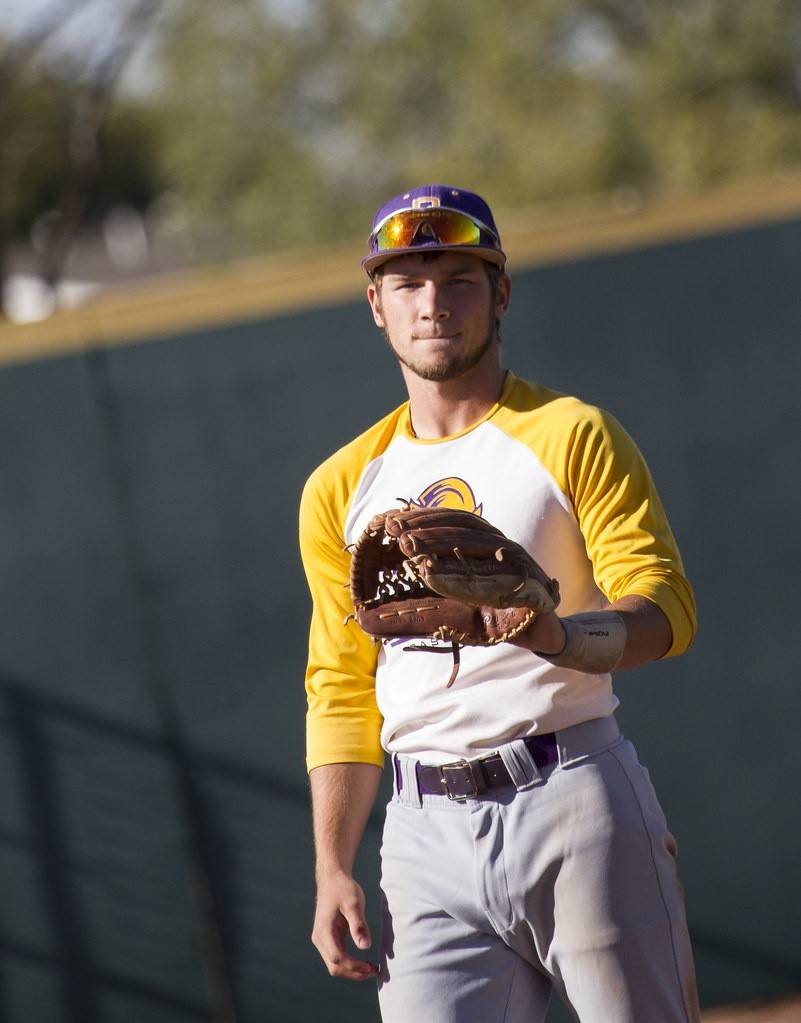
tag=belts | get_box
[396,731,560,802]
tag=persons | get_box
[297,183,707,1023]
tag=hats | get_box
[360,185,506,282]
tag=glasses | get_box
[367,207,499,250]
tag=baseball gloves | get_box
[349,506,562,647]
